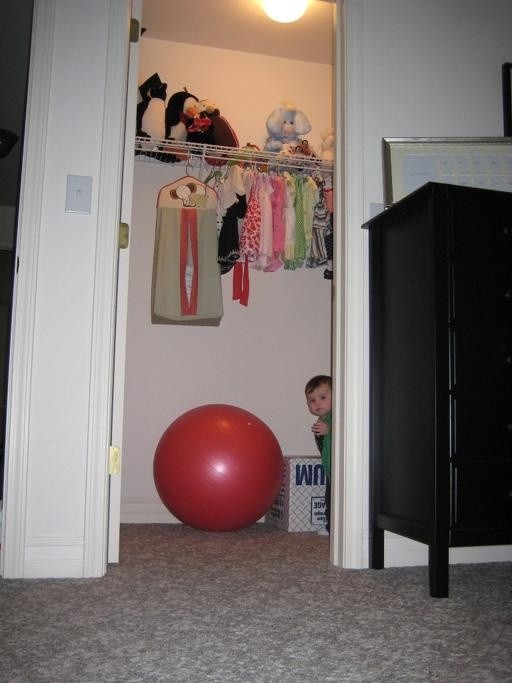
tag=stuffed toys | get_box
[136,73,335,174]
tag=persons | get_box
[306,374,329,535]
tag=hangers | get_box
[172,155,332,186]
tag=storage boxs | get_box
[266,453,327,533]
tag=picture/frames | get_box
[380,135,512,209]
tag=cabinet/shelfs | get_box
[362,176,512,600]
